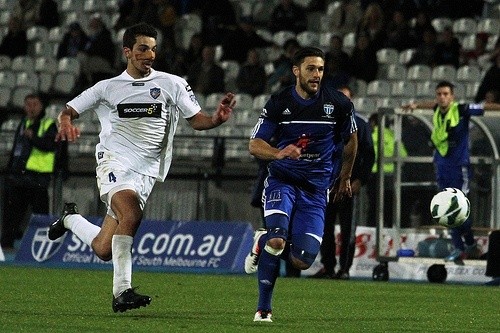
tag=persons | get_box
[1,0,500,321]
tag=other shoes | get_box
[464,241,478,258]
[313,267,349,280]
[445,249,466,262]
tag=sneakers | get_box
[112,288,152,313]
[254,309,273,323]
[244,227,267,274]
[48,202,79,240]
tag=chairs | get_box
[0,0,500,163]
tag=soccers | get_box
[430,187,470,227]
